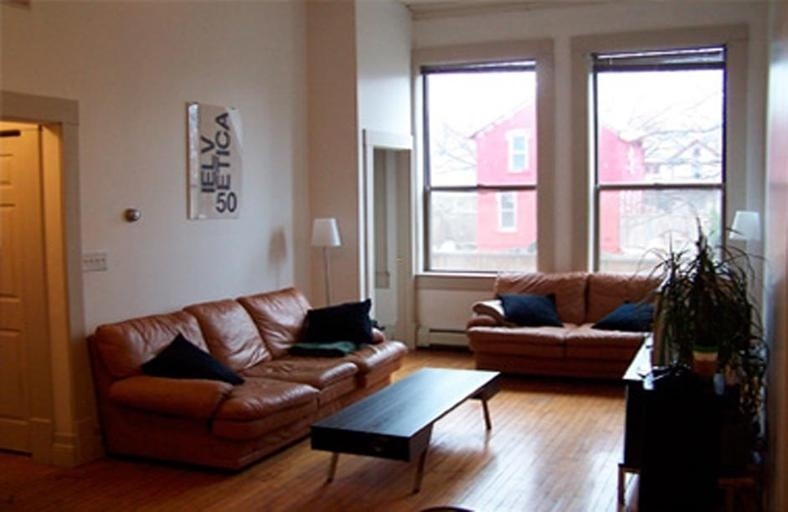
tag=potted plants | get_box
[629,230,771,407]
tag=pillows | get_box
[286,341,356,358]
[140,332,246,386]
[297,299,373,343]
[472,299,516,326]
[591,299,657,333]
[496,292,566,327]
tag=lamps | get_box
[728,210,761,296]
[309,217,343,307]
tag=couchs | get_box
[465,271,662,381]
[85,286,407,472]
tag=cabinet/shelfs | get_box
[618,331,741,512]
